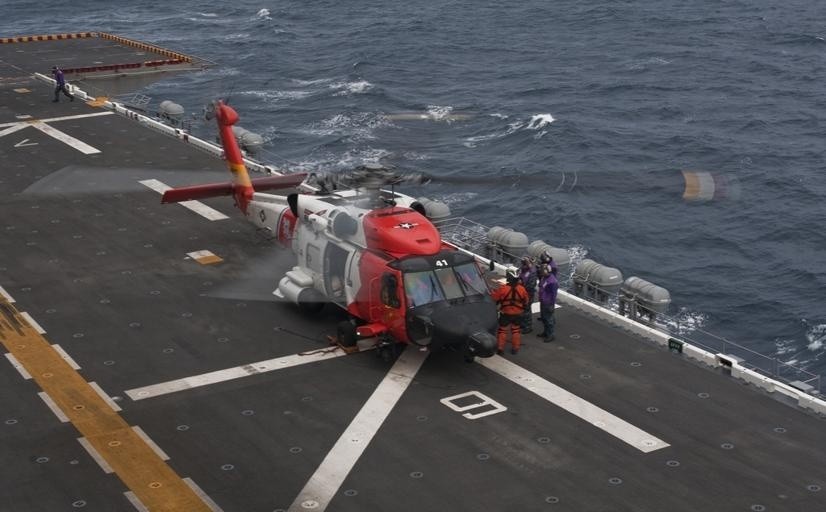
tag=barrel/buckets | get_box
[160,101,185,121]
[622,276,671,313]
[487,225,527,256]
[576,259,623,291]
[415,197,450,218]
[528,239,570,271]
[216,126,264,153]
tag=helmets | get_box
[521,250,551,267]
[506,265,519,280]
[537,263,552,275]
[50,65,59,74]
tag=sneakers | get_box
[497,347,521,356]
[522,326,534,335]
[538,315,554,343]
[52,96,75,103]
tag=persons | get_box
[488,269,529,356]
[533,251,559,324]
[516,255,538,334]
[535,262,559,344]
[50,65,74,102]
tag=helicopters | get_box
[19,99,745,362]
